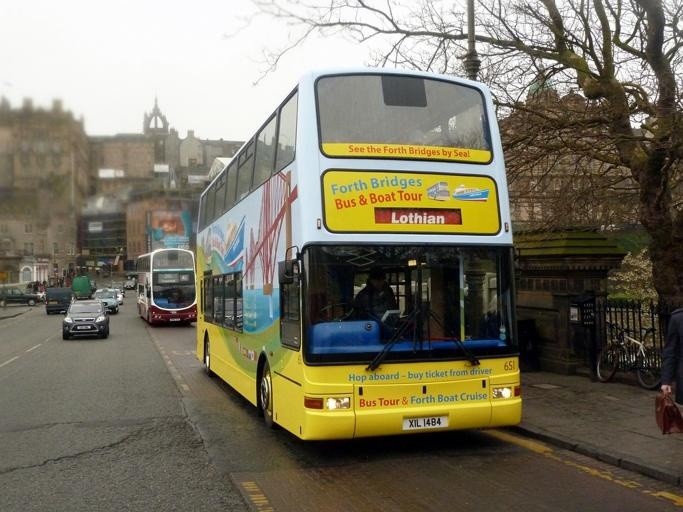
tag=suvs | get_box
[90,281,97,292]
[111,282,125,298]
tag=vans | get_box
[45,276,94,315]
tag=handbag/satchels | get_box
[655,392,682,435]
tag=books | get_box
[380,309,401,322]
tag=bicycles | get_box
[596,321,662,390]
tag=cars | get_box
[0,286,40,307]
[63,288,123,340]
[124,280,135,290]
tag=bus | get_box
[136,248,197,325]
[197,70,522,440]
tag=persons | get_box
[350,267,412,341]
[659,304,683,406]
